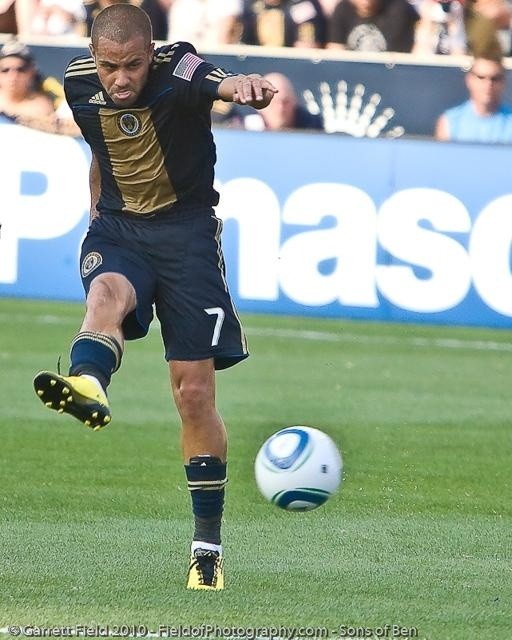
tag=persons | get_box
[34,4,279,592]
[0,0,512,143]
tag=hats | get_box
[1,41,34,63]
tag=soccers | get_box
[255,426,345,510]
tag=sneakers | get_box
[33,372,114,432]
[188,544,226,594]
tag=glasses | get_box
[0,66,30,75]
